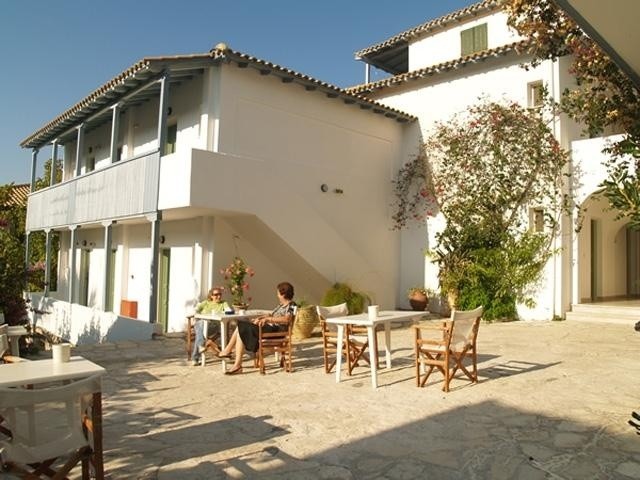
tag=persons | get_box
[192,287,232,366]
[215,282,298,375]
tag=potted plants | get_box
[407,287,431,311]
[291,299,316,338]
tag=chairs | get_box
[412,306,483,392]
[317,305,379,376]
[187,316,233,361]
[0,375,105,479]
[3,355,72,448]
[254,305,297,374]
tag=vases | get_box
[232,301,250,315]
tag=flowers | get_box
[220,256,255,305]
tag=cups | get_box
[367,305,380,319]
[51,343,71,363]
[239,309,246,315]
[211,309,217,315]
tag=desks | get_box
[0,354,108,386]
[195,309,281,374]
[7,326,27,357]
[326,310,430,388]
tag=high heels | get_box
[214,352,232,358]
[225,365,242,375]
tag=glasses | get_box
[212,292,221,296]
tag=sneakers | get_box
[193,360,199,366]
[199,346,206,353]
